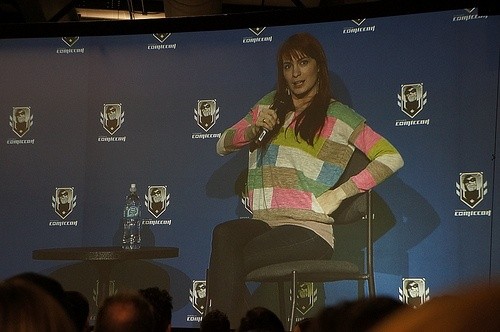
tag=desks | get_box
[31,247,180,326]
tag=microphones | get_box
[255,92,293,147]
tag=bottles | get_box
[122,184,143,249]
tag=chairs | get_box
[244,149,377,332]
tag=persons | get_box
[298,279,500,332]
[239,307,285,332]
[207,34,404,316]
[200,309,230,332]
[62,291,90,332]
[0,273,65,332]
[142,288,173,332]
[94,291,159,332]
